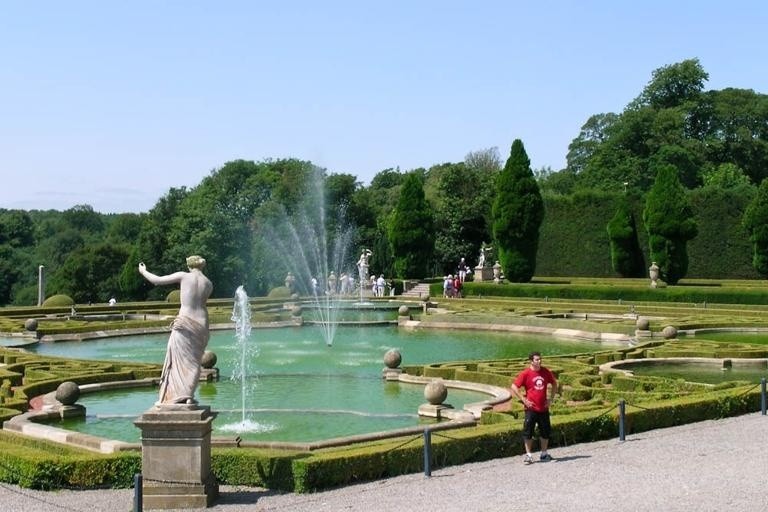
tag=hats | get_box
[443,274,459,279]
[461,258,465,263]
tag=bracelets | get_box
[550,395,554,398]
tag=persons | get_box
[511,351,557,464]
[357,248,374,276]
[108,295,117,305]
[138,255,215,404]
[285,270,396,298]
[443,258,466,298]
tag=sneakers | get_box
[539,453,558,463]
[523,455,535,464]
